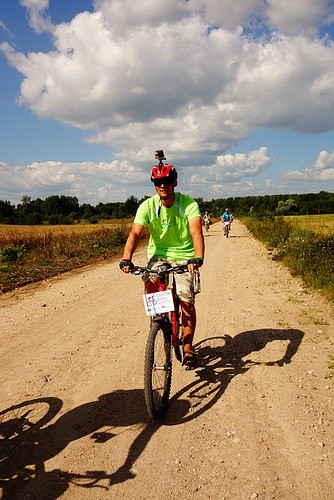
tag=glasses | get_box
[153,179,174,187]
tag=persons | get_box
[203,211,210,230]
[120,164,205,368]
[221,208,232,235]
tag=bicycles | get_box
[124,262,196,417]
[223,222,230,238]
[203,218,210,232]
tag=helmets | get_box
[205,211,208,213]
[225,208,230,211]
[150,163,177,183]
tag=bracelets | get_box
[195,257,203,259]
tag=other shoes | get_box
[183,350,195,364]
